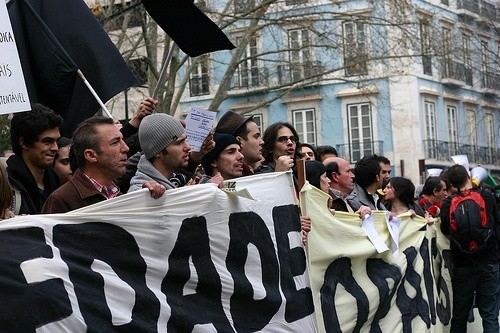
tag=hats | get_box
[292,161,329,181]
[216,110,255,134]
[139,113,186,160]
[201,134,241,168]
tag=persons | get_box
[415,176,448,218]
[347,155,434,226]
[440,164,500,333]
[0,103,63,220]
[52,137,75,185]
[118,97,311,246]
[41,117,129,214]
[293,143,372,220]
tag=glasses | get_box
[274,136,298,144]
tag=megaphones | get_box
[470,166,488,188]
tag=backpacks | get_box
[448,187,495,258]
